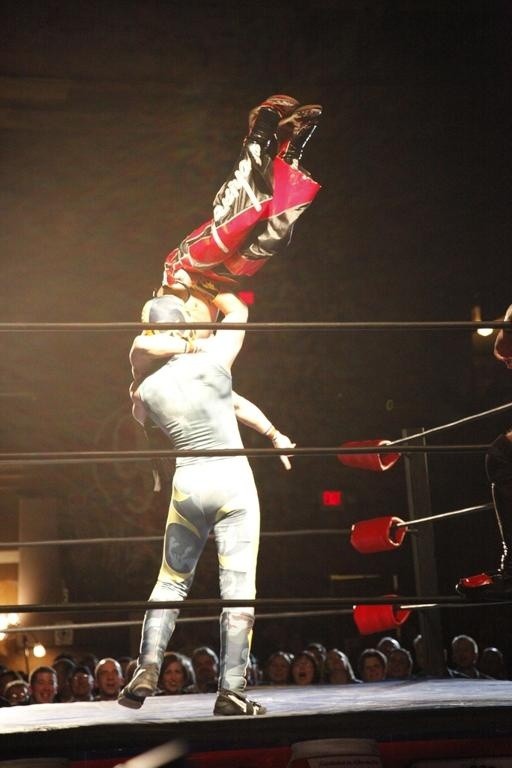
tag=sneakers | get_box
[214,689,266,715]
[117,667,160,709]
[248,94,323,142]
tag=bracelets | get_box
[263,421,280,442]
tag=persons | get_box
[118,288,269,717]
[1,631,509,710]
[129,93,324,492]
